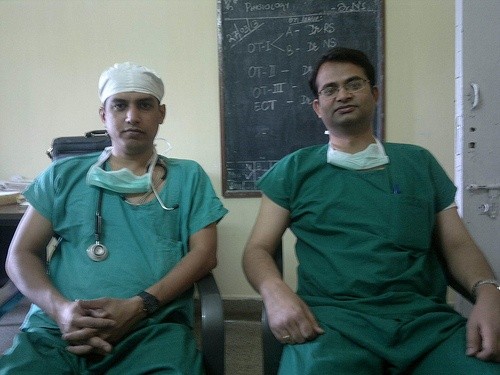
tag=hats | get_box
[98,62,164,105]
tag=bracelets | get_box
[471,279,500,302]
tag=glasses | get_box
[317,78,370,97]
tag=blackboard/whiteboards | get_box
[216,0,385,199]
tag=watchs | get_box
[136,290,161,316]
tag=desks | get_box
[0,202,35,230]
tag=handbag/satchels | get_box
[46,129,112,162]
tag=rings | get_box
[281,335,290,339]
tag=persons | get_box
[0,62,230,375]
[242,47,500,375]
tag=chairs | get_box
[259,220,496,375]
[1,249,225,375]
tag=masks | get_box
[87,143,158,194]
[325,130,389,170]
